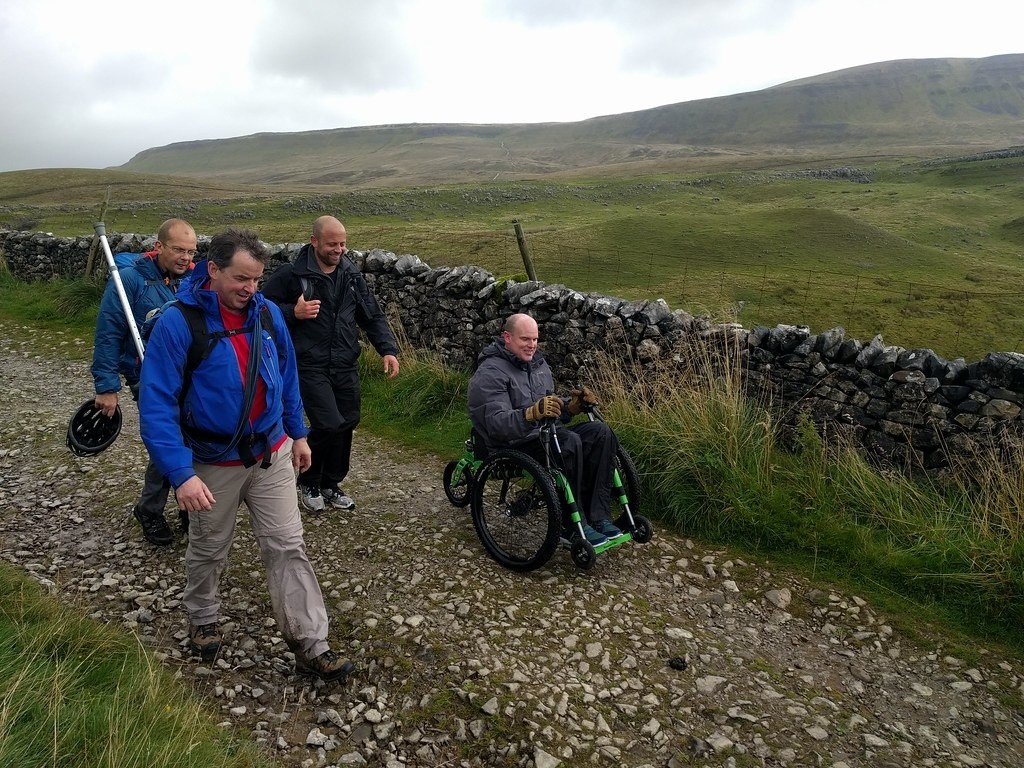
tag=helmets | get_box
[66,399,122,457]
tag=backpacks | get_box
[131,299,279,469]
[113,252,184,355]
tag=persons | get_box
[90,218,199,546]
[140,228,355,682]
[466,313,624,548]
[258,215,400,513]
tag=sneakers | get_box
[299,484,355,512]
[560,519,623,547]
[178,509,189,535]
[189,622,222,654]
[295,650,355,682]
[134,503,173,546]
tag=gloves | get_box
[567,386,598,417]
[526,396,564,422]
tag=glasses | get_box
[160,241,198,256]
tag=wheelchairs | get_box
[443,381,656,575]
